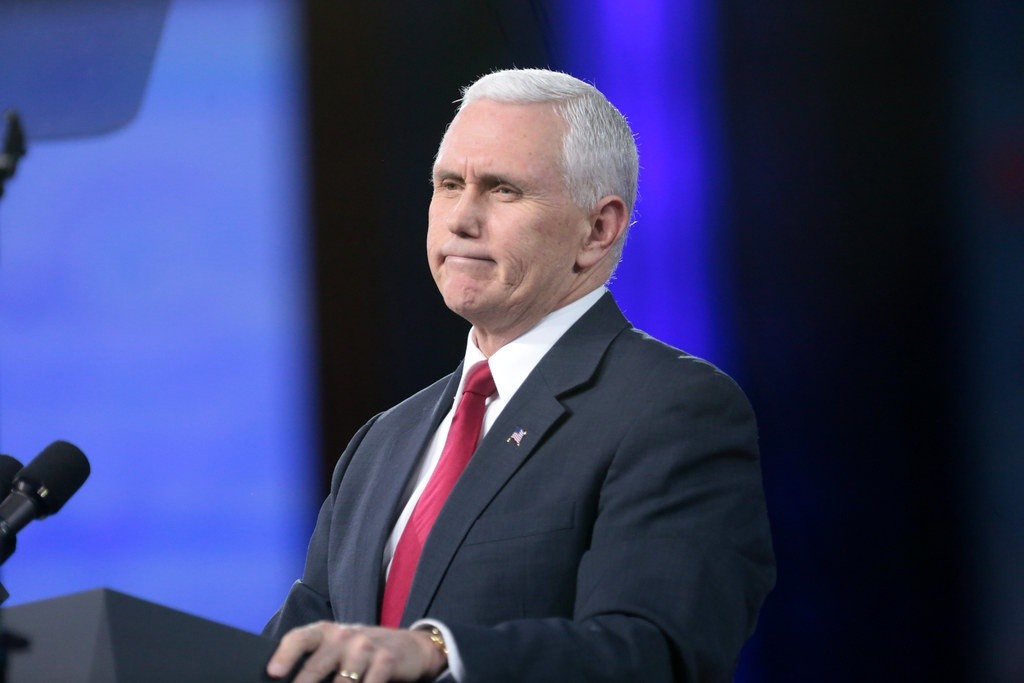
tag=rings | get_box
[337,668,361,682]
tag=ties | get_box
[378,359,497,629]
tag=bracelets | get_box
[429,625,448,657]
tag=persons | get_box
[259,67,773,683]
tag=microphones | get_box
[0,441,91,566]
